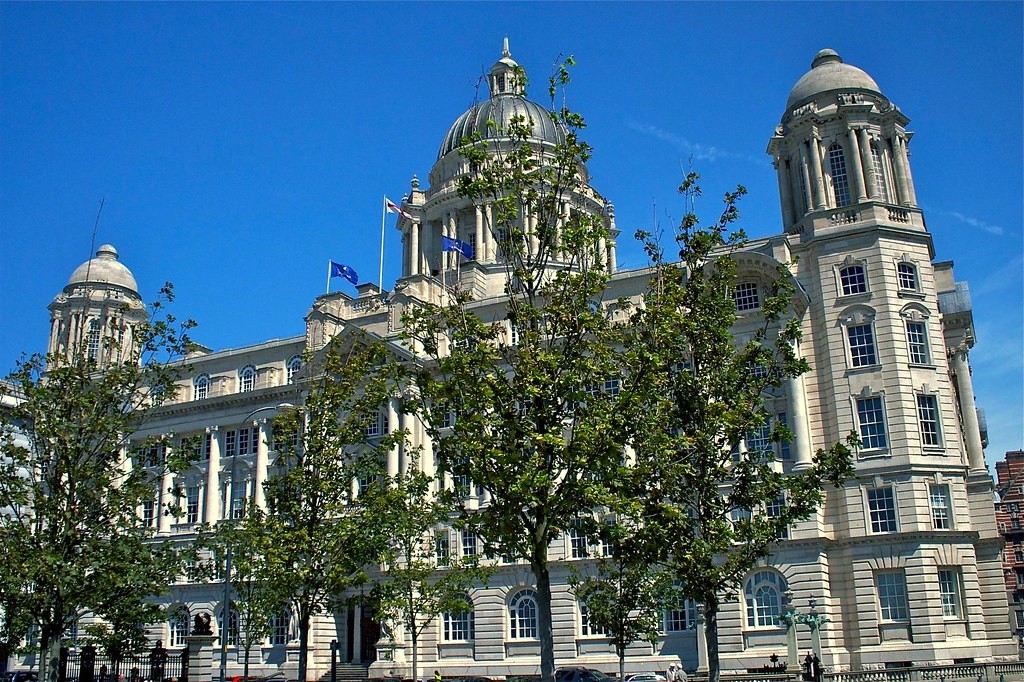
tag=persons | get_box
[675,663,687,682]
[434,670,441,680]
[666,663,676,682]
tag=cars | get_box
[553,666,665,682]
[1,671,39,682]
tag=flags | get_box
[442,236,472,258]
[385,198,420,223]
[330,262,358,285]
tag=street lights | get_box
[329,640,340,682]
[220,403,294,681]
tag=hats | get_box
[670,663,675,667]
[677,664,682,668]
